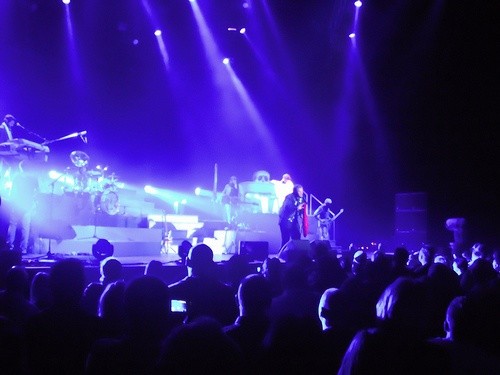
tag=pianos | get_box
[0,138,50,197]
[238,170,282,214]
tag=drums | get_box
[93,190,120,216]
[61,191,91,209]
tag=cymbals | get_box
[103,181,125,190]
[86,171,102,179]
[70,151,90,168]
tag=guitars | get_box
[318,209,344,239]
[221,197,260,209]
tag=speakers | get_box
[238,240,269,262]
[393,192,430,251]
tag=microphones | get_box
[303,197,308,204]
[16,122,24,128]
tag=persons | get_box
[0,114,15,171]
[313,197,333,241]
[276,174,293,209]
[5,159,41,264]
[0,237,500,375]
[279,184,306,253]
[221,176,242,225]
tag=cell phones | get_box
[169,299,188,312]
